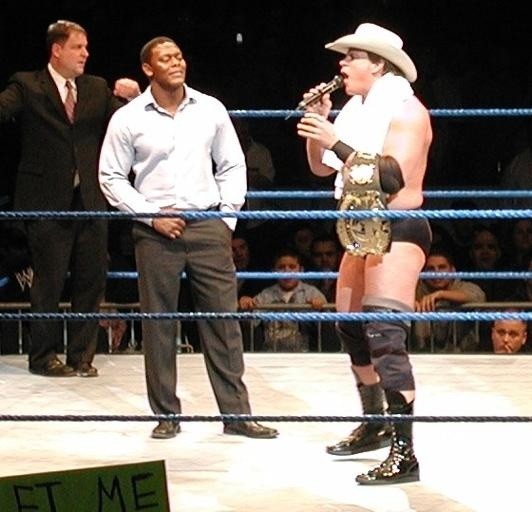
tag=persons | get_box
[99,36,280,439]
[297,23,434,486]
[1,187,532,356]
[0,20,140,377]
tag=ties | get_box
[64,82,78,126]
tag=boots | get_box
[325,382,391,456]
[355,391,420,486]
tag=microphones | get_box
[296,73,345,111]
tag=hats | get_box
[324,22,418,84]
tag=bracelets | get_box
[328,140,355,166]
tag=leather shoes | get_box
[29,359,74,377]
[151,423,181,439]
[74,366,99,378]
[223,422,281,439]
